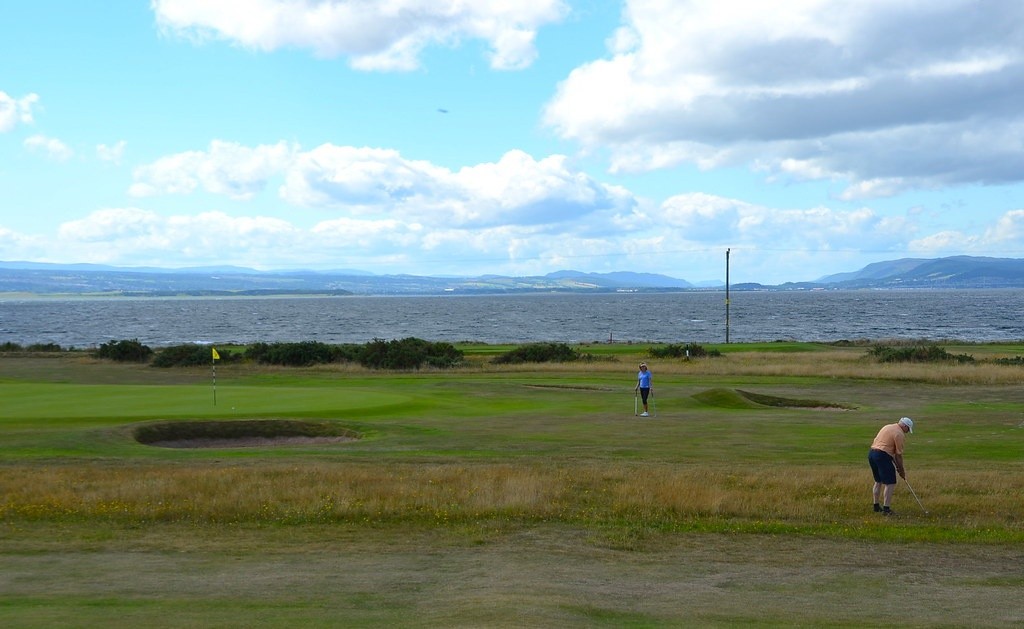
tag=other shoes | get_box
[873,506,884,513]
[882,509,898,517]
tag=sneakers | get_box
[640,412,649,417]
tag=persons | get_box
[867,416,916,514]
[634,362,653,417]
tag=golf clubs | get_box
[903,477,928,513]
[634,388,638,416]
[651,390,656,418]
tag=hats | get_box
[901,417,912,434]
